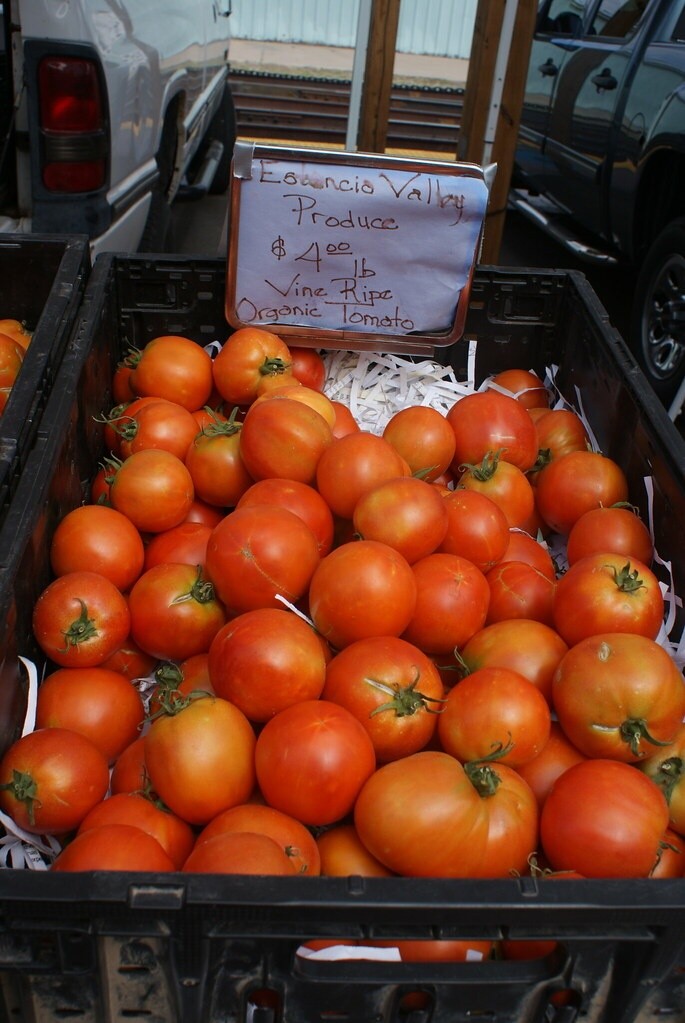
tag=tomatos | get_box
[0,314,685,1016]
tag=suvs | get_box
[501,0,684,419]
[0,0,239,332]
